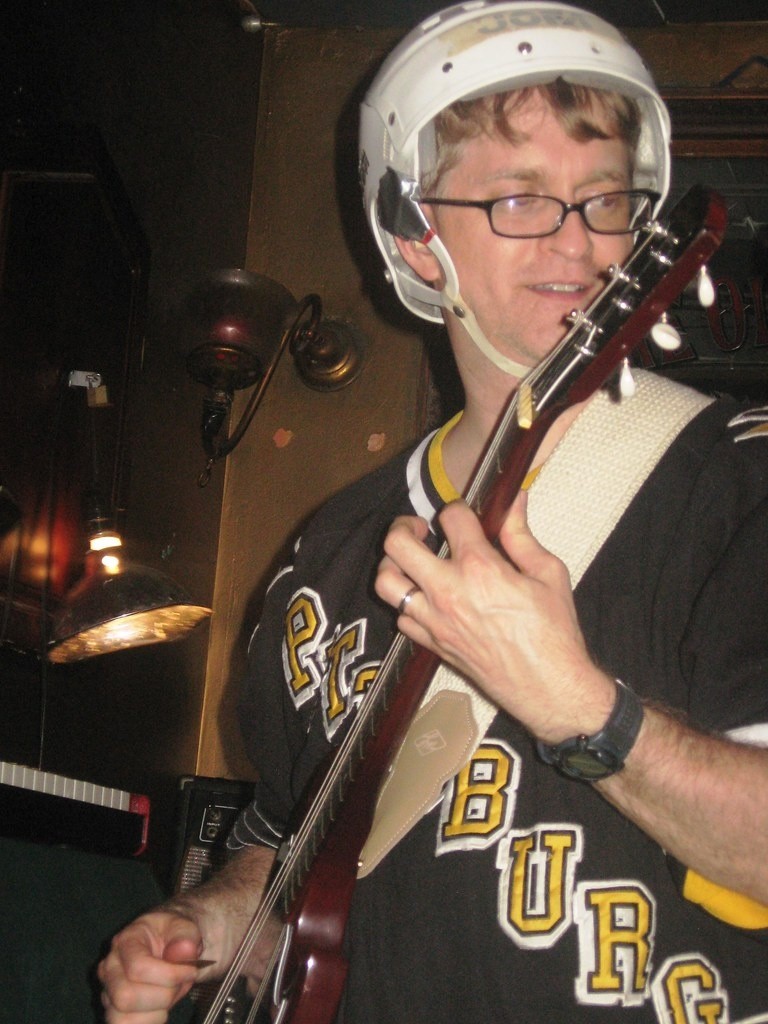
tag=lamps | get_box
[47,368,213,672]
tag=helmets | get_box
[357,0,668,321]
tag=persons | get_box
[97,1,766,1023]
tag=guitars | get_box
[170,179,737,1023]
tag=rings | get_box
[396,586,422,614]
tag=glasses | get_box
[422,185,663,236]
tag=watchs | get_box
[535,675,645,784]
